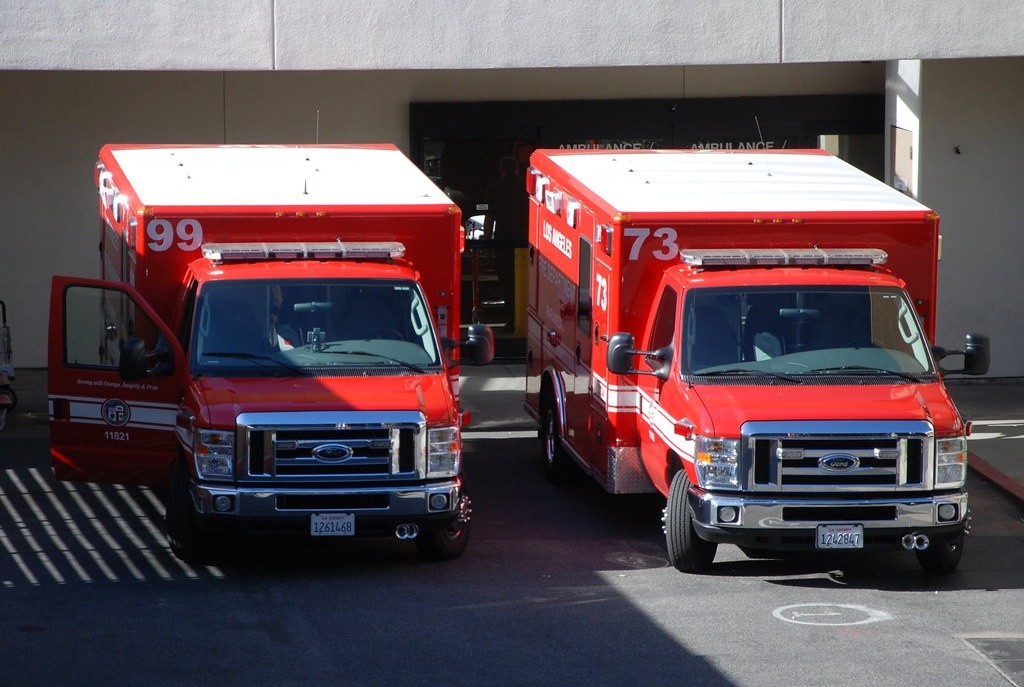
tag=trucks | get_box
[48,144,494,566]
[523,148,989,576]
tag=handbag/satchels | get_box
[277,323,301,352]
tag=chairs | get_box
[692,306,867,371]
[202,292,400,353]
